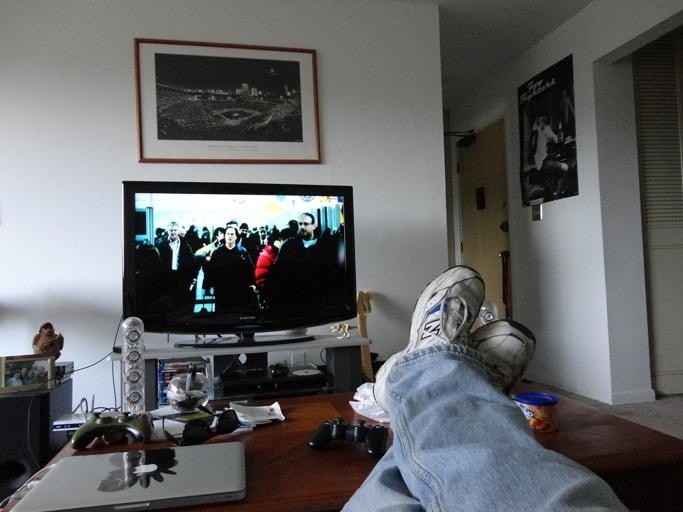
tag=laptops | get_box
[9,441,247,512]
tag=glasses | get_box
[163,405,240,446]
[299,222,308,225]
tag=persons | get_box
[6,365,48,387]
[530,113,569,196]
[336,264,627,512]
[136,212,346,319]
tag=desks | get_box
[1,380,682,512]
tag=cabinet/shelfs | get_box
[0,377,73,503]
[110,337,372,408]
[634,39,682,398]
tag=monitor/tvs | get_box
[122,181,357,348]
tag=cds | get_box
[292,369,320,375]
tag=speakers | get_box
[326,345,363,392]
[120,317,145,418]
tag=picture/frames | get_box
[133,38,320,165]
[0,353,55,393]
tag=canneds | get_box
[516,391,559,434]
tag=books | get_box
[150,358,212,424]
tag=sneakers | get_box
[373,265,535,413]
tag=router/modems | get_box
[52,396,96,432]
